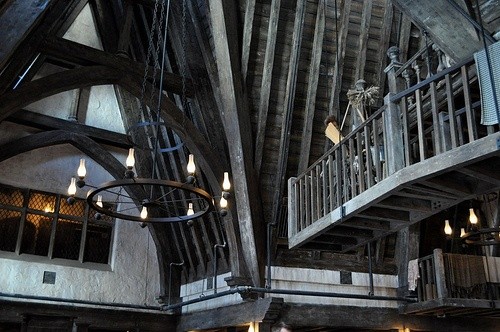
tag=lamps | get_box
[440,178,500,247]
[61,0,234,226]
